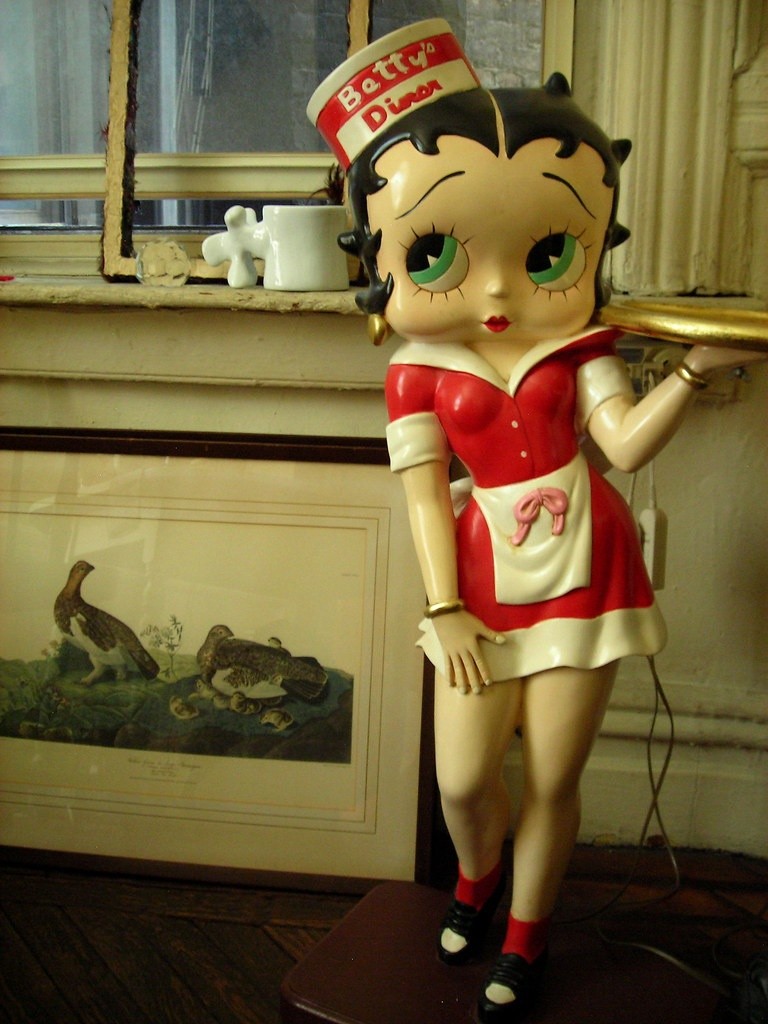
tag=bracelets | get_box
[423,599,466,619]
[676,363,709,392]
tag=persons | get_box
[305,16,768,1024]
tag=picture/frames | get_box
[0,426,454,895]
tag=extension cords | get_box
[639,508,667,591]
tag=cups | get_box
[200,203,350,290]
